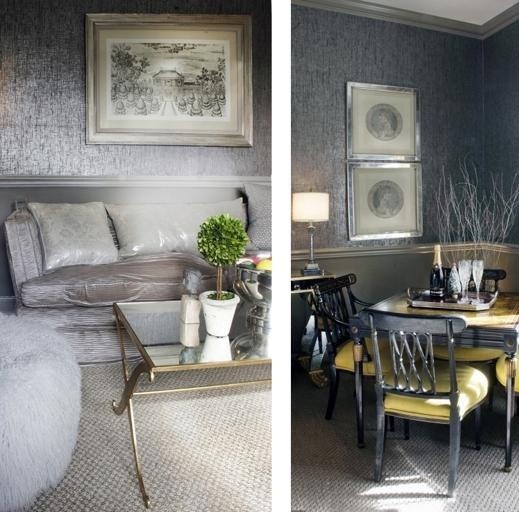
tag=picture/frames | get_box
[344,80,423,161]
[82,11,254,148]
[346,160,425,242]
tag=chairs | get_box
[427,267,506,412]
[314,274,419,431]
[495,350,519,400]
[359,308,489,500]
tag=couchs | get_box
[4,199,272,367]
[0,311,83,511]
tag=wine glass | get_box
[471,259,486,304]
[457,258,471,304]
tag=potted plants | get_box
[193,212,251,339]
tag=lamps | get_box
[291,192,331,276]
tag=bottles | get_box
[448,263,461,300]
[429,244,447,296]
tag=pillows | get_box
[104,195,244,262]
[27,200,119,274]
[242,183,272,252]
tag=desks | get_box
[291,268,335,354]
